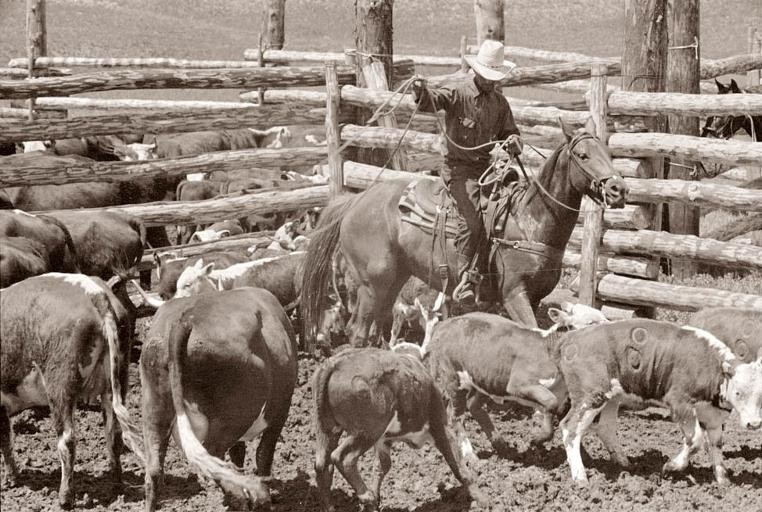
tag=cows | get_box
[306,272,762,510]
[0,115,328,512]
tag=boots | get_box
[457,254,475,301]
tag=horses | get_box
[297,115,629,362]
[700,78,762,142]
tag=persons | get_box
[410,40,523,307]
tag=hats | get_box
[463,39,517,82]
